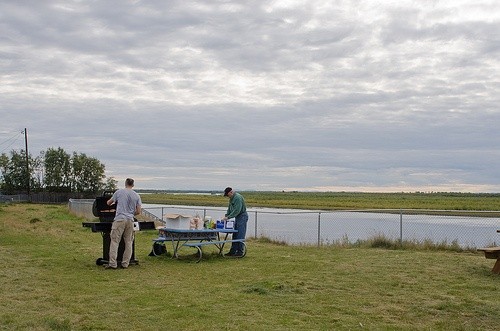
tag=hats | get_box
[223,186,233,197]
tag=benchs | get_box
[182,239,247,264]
[154,237,217,259]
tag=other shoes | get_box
[225,251,235,257]
[121,265,128,268]
[235,251,244,258]
[105,265,118,270]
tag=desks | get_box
[159,228,238,263]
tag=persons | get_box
[103,178,141,270]
[222,187,248,255]
